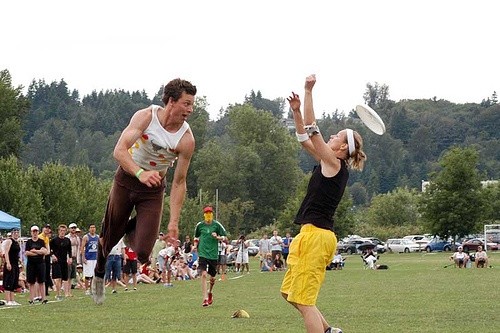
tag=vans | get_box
[231,239,259,255]
[385,238,420,253]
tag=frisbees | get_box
[356,102,386,136]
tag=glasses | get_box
[12,229,20,231]
[76,231,80,233]
[71,227,76,229]
[19,267,22,269]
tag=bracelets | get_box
[221,235,226,242]
[304,121,320,138]
[135,168,144,178]
[194,237,199,243]
[295,130,309,143]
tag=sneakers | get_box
[42,299,48,305]
[134,288,137,290]
[208,293,213,304]
[124,288,128,290]
[91,276,105,305]
[24,288,29,293]
[203,298,209,306]
[38,296,42,301]
[27,299,35,306]
[33,297,39,302]
[112,290,117,294]
[168,283,173,287]
[12,301,22,305]
[324,326,343,333]
[7,300,18,306]
[162,283,169,287]
[85,289,91,296]
[122,235,132,248]
[156,279,159,283]
[159,278,162,281]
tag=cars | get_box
[463,237,498,250]
[402,234,425,240]
[336,238,384,253]
[415,235,440,251]
[425,237,461,252]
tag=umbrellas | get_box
[357,241,377,250]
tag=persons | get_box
[82,224,99,297]
[262,253,275,272]
[193,205,228,307]
[64,223,77,298]
[227,237,251,274]
[35,223,52,301]
[475,246,490,269]
[1,231,12,262]
[75,228,82,264]
[274,253,285,271]
[449,246,468,269]
[44,230,55,295]
[258,233,269,271]
[105,232,176,293]
[71,264,85,289]
[49,224,73,300]
[280,74,367,333]
[218,241,227,275]
[326,250,347,271]
[90,79,198,306]
[362,248,377,270]
[25,225,48,305]
[18,263,29,293]
[4,227,22,306]
[271,229,283,261]
[176,236,199,280]
[282,229,293,268]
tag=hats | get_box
[7,232,11,235]
[180,255,184,258]
[76,228,82,231]
[458,246,463,249]
[31,226,39,231]
[159,232,163,234]
[69,223,77,228]
[478,246,482,249]
[188,254,193,257]
[42,224,51,227]
[204,207,213,212]
[194,237,199,242]
